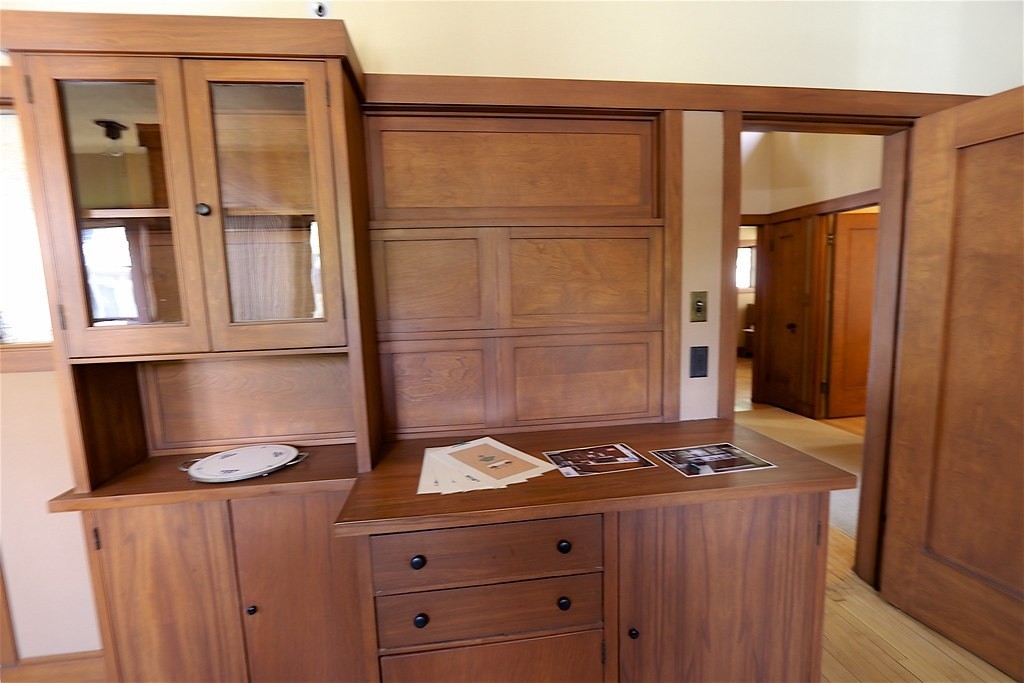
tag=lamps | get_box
[92,120,130,157]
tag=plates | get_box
[178,444,308,481]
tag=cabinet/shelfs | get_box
[0,8,860,683]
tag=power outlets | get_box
[690,290,707,323]
